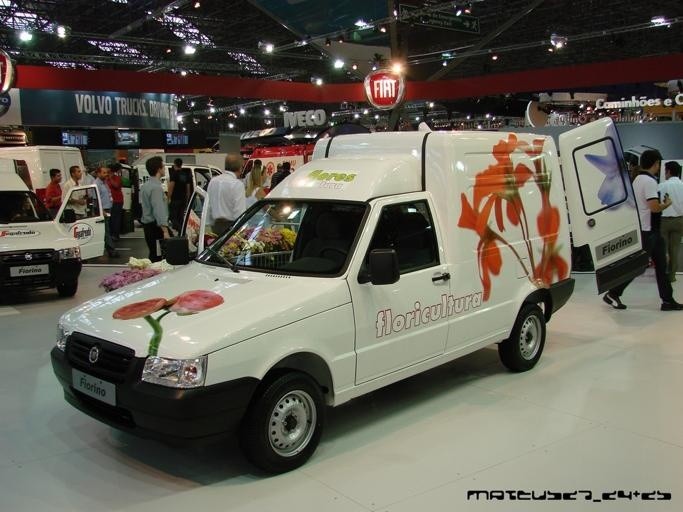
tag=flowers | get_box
[99,225,299,291]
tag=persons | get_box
[602,150,683,310]
[12,151,293,263]
[657,160,683,282]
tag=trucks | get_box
[50,116,650,478]
[0,145,229,306]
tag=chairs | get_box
[301,212,349,267]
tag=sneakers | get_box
[107,248,119,258]
[603,292,627,309]
[661,303,683,311]
